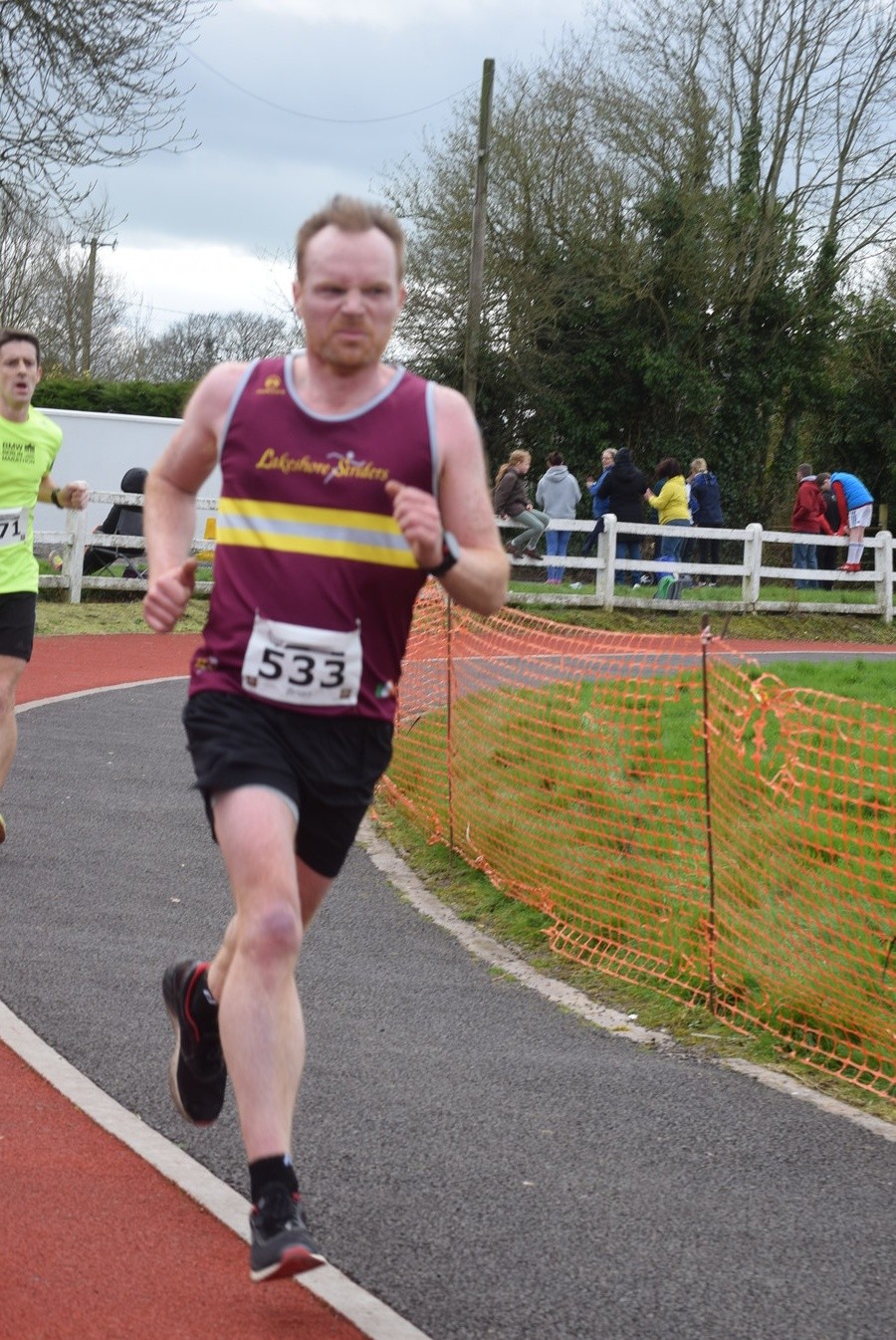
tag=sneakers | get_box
[544,579,562,585]
[163,956,228,1125]
[250,1181,326,1282]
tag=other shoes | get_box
[836,563,860,571]
[522,547,543,560]
[505,540,524,559]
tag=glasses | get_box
[601,457,613,460]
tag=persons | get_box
[586,448,644,591]
[491,450,551,561]
[140,191,511,1283]
[0,323,89,851]
[790,463,875,592]
[48,467,148,576]
[536,448,583,584]
[688,458,724,590]
[645,458,688,604]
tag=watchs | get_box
[429,530,460,577]
[50,487,64,509]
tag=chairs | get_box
[86,508,149,599]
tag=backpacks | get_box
[653,576,680,616]
